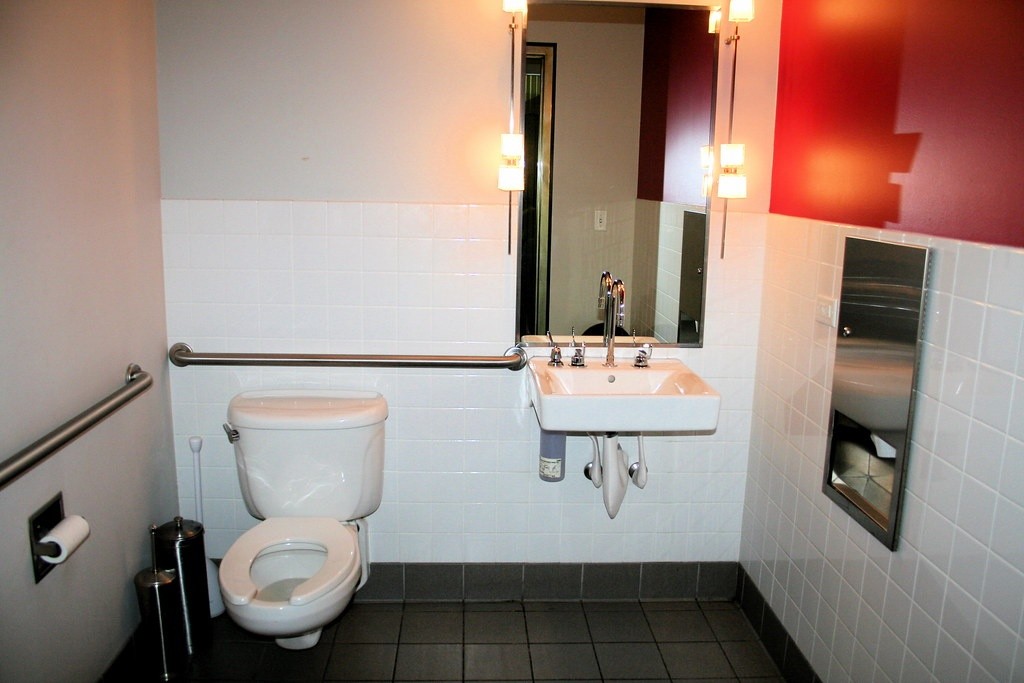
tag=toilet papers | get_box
[40,515,90,563]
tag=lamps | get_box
[498,0,524,255]
[718,0,756,259]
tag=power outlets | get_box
[595,211,607,230]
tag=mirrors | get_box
[515,0,720,348]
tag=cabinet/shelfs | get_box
[637,6,714,206]
[677,211,706,343]
[822,235,933,552]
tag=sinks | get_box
[528,357,721,433]
[521,335,663,342]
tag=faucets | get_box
[597,271,614,344]
[606,280,625,366]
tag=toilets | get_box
[219,395,384,651]
